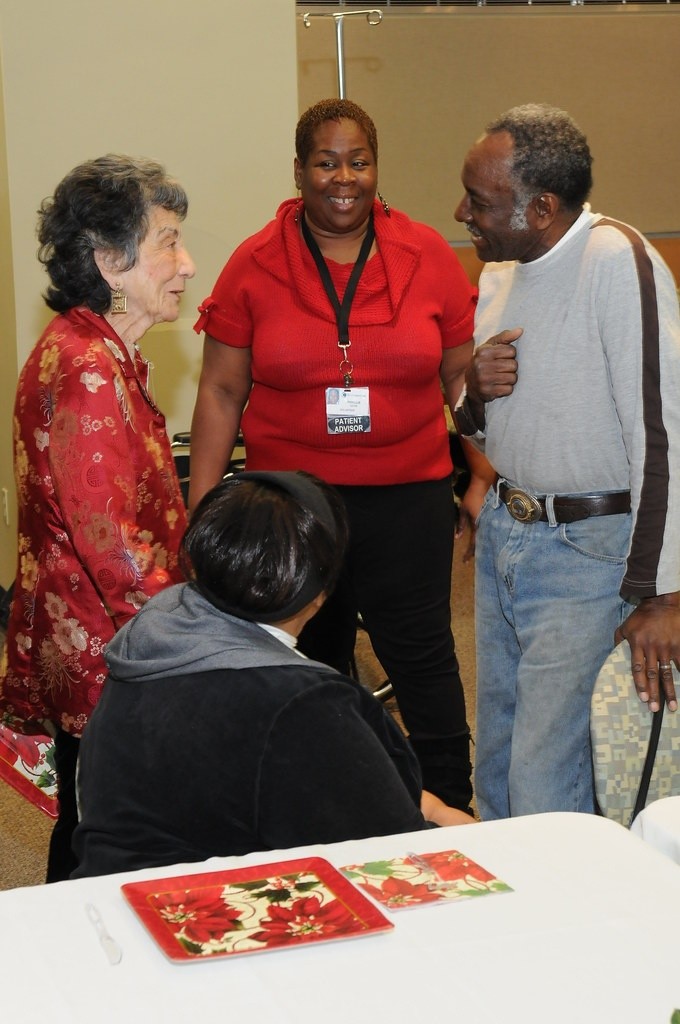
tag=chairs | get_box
[589,639,680,831]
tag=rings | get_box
[660,665,672,670]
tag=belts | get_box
[492,476,630,523]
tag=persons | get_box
[327,389,338,404]
[453,101,680,821]
[186,97,497,819]
[5,151,199,884]
[68,468,478,882]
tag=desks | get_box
[0,810,680,1024]
